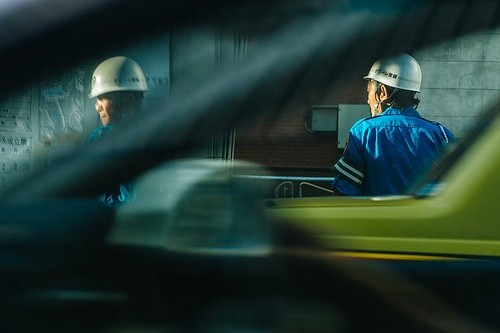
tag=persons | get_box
[329,54,461,197]
[68,56,154,209]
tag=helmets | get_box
[360,53,422,94]
[88,54,150,101]
